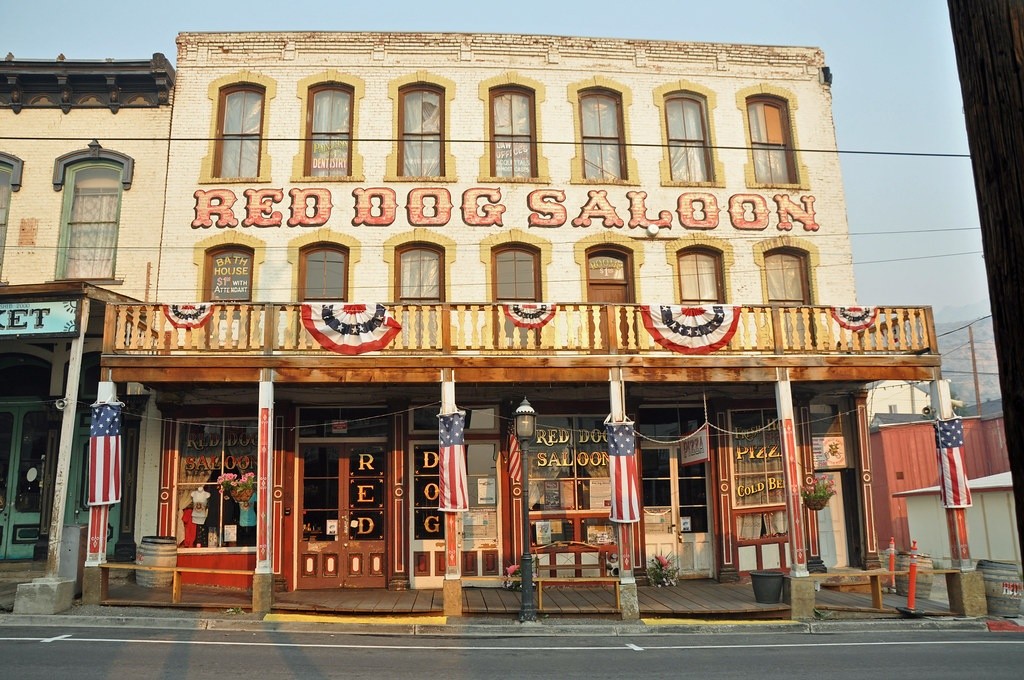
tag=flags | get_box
[439,414,469,511]
[606,425,641,521]
[933,419,972,505]
[89,405,121,502]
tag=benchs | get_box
[98,562,253,607]
[810,569,961,609]
[461,576,621,610]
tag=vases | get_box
[216,472,255,500]
[229,488,254,502]
[647,567,680,587]
[507,575,523,590]
[802,498,828,511]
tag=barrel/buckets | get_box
[976,560,1022,617]
[750,571,785,603]
[895,550,933,599]
[135,536,178,588]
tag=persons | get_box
[191,487,210,524]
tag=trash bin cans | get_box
[135,536,178,586]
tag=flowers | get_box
[646,550,678,570]
[506,565,523,577]
[800,474,838,501]
